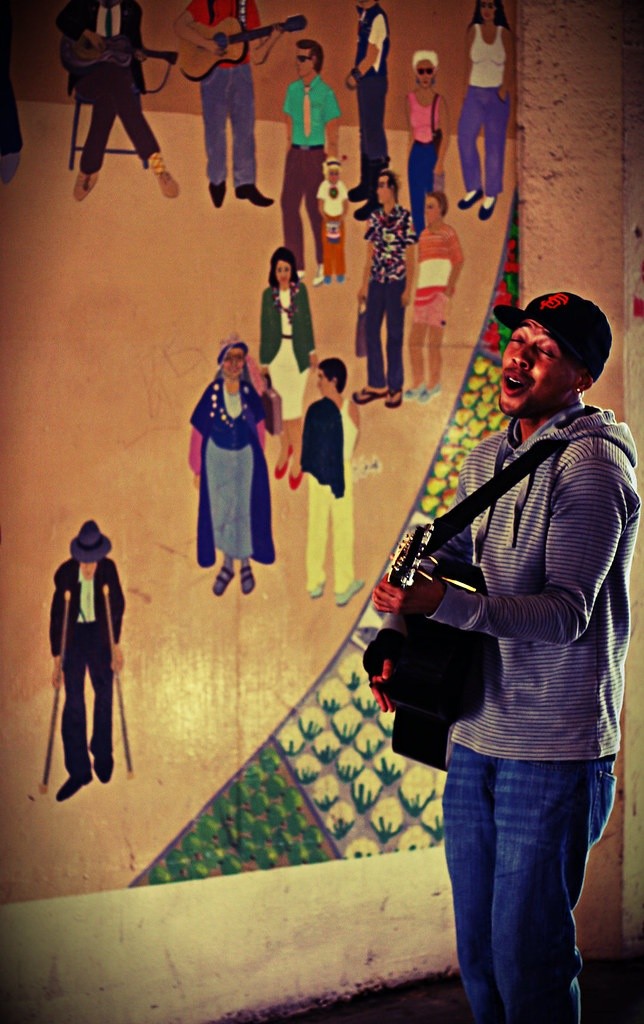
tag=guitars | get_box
[375,522,480,773]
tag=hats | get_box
[493,291,613,385]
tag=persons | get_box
[370,290,641,1024]
